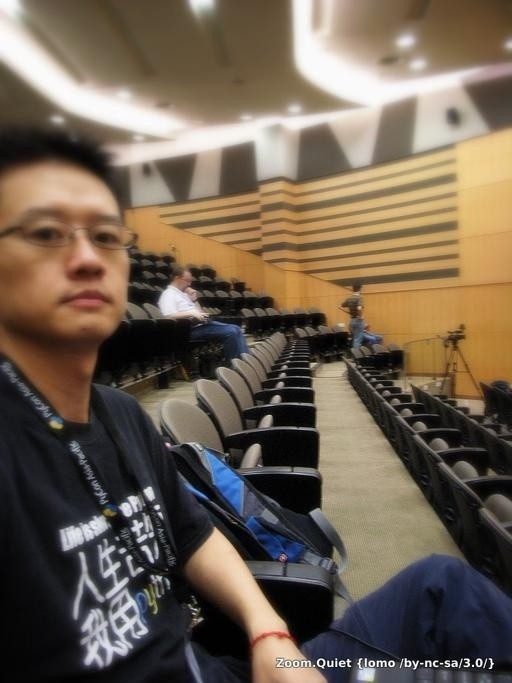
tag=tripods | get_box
[436,344,485,402]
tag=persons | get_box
[157,266,249,366]
[348,319,384,348]
[338,283,364,349]
[0,123,512,682]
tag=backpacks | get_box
[170,440,348,576]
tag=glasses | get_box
[1,219,139,250]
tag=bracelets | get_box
[249,631,293,648]
[193,299,198,302]
